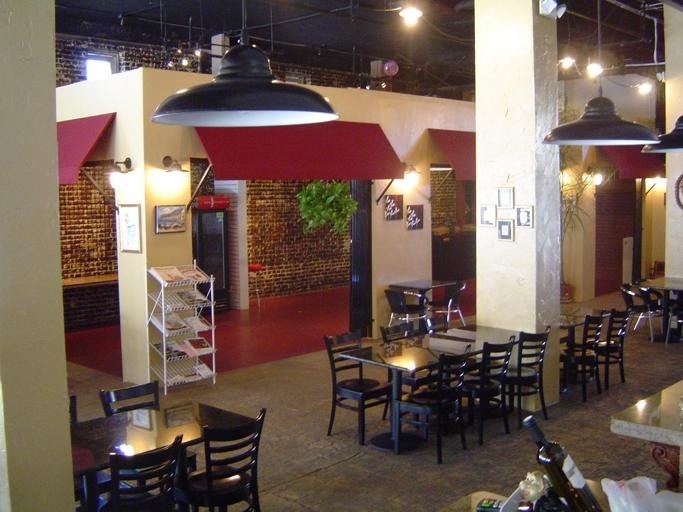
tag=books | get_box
[151,266,213,378]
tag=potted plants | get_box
[557,107,618,304]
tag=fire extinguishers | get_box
[190,194,230,210]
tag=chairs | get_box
[323,314,551,464]
[663,289,683,343]
[629,279,663,344]
[69,379,268,512]
[379,288,431,343]
[425,280,468,335]
[619,284,650,335]
[586,307,632,391]
[559,309,616,402]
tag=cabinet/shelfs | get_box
[147,257,218,396]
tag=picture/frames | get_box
[117,203,142,253]
[479,186,534,242]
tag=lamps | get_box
[542,1,662,147]
[641,113,683,153]
[150,1,339,128]
[103,157,131,174]
[162,156,189,173]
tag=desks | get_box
[387,280,456,333]
[560,307,611,384]
[638,276,683,343]
[609,377,683,493]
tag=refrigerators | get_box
[191,194,231,315]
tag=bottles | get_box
[210,196,213,207]
[519,413,604,512]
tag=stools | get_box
[247,255,267,309]
